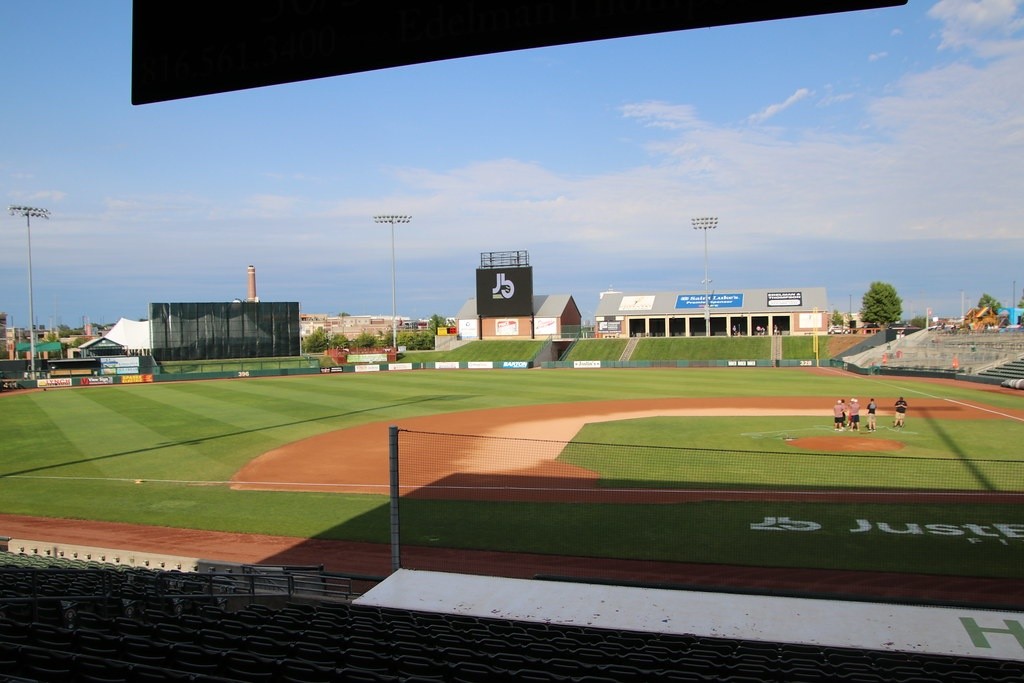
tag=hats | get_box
[854,400,857,402]
[838,400,841,403]
[851,398,854,401]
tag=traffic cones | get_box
[882,352,887,363]
[952,354,958,369]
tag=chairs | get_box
[0,552,1024,683]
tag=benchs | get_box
[979,358,1024,379]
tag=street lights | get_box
[6,205,51,380]
[374,215,412,348]
[691,216,720,336]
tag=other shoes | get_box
[834,425,876,433]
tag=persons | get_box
[834,398,861,432]
[733,325,736,335]
[894,397,907,427]
[773,325,778,335]
[867,398,877,431]
[756,326,765,336]
